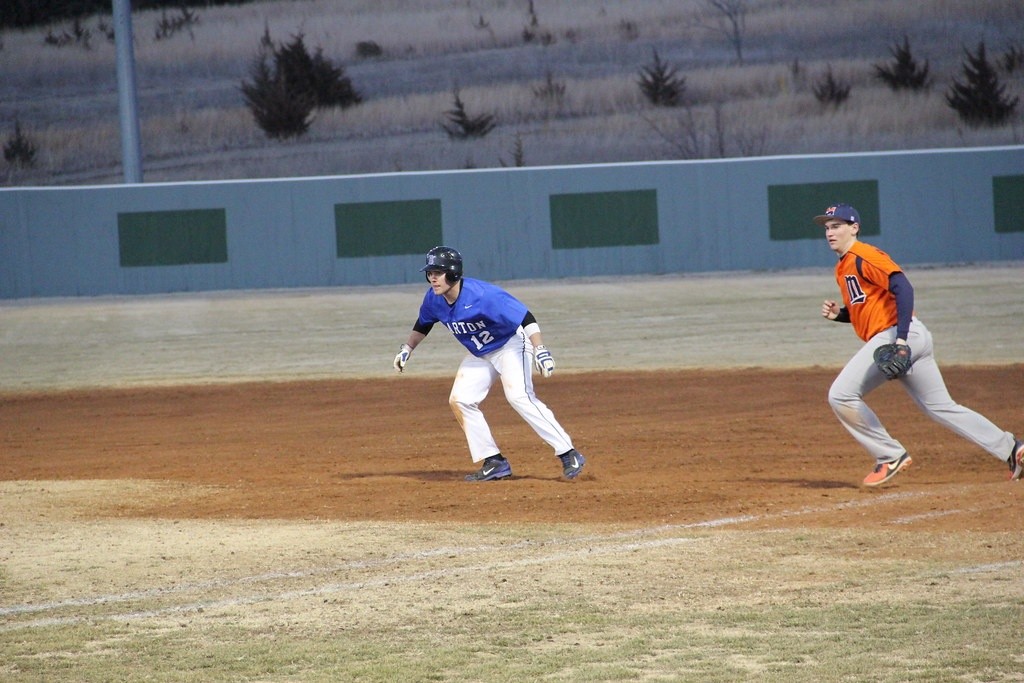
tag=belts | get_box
[893,319,912,326]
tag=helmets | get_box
[418,246,463,284]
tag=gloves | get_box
[393,344,411,374]
[534,345,556,379]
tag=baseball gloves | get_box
[873,342,912,381]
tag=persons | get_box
[814,203,1024,485]
[394,246,585,479]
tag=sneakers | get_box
[1008,438,1024,481]
[465,457,512,481]
[862,451,913,486]
[560,448,585,478]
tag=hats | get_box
[813,205,861,228]
